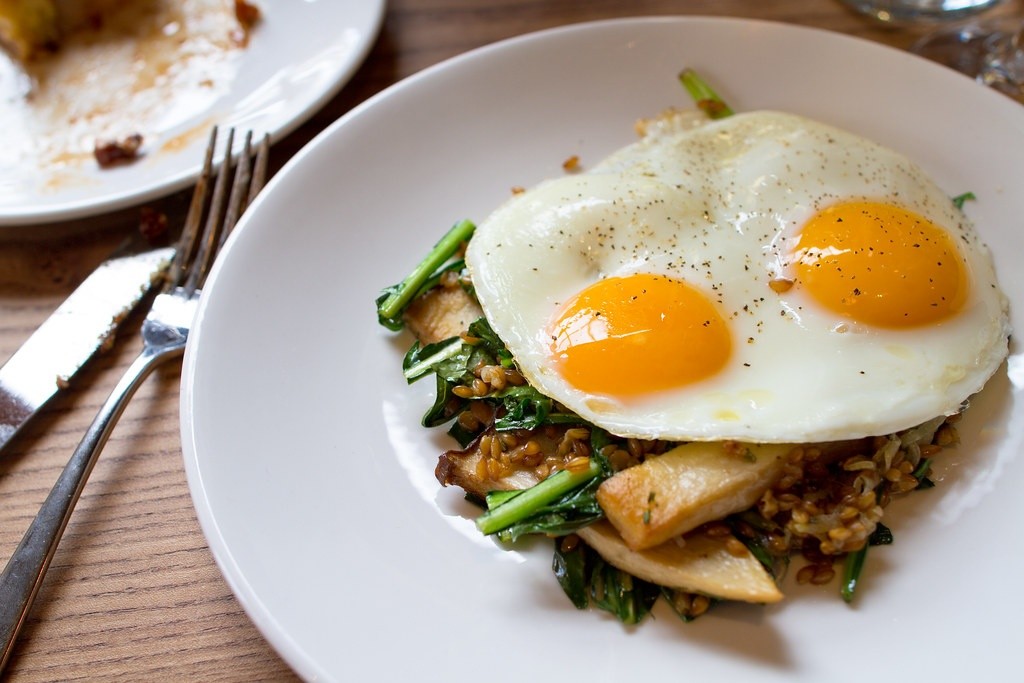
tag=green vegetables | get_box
[377,218,663,626]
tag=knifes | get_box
[1,193,208,452]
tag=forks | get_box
[0,125,268,675]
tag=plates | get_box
[179,13,1023,683]
[0,0,388,226]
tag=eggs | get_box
[465,109,1010,442]
